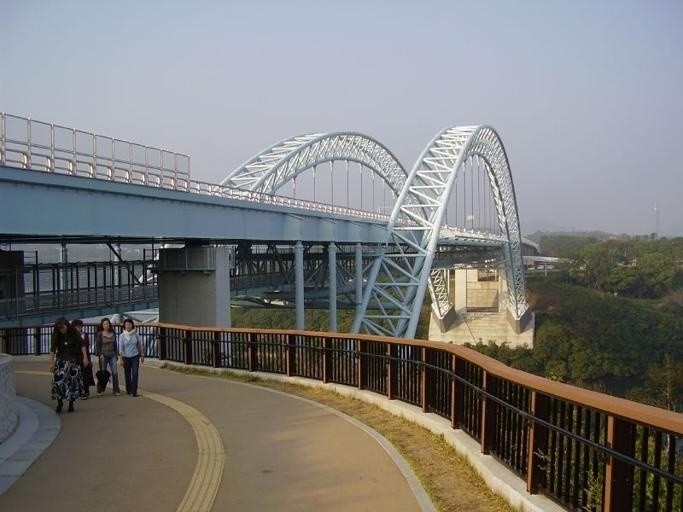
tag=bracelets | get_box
[140,354,144,356]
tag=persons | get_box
[47,317,90,415]
[117,318,144,397]
[68,319,95,401]
[93,317,121,397]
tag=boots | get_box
[56,401,75,413]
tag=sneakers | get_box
[80,392,139,400]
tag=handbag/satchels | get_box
[76,352,83,365]
